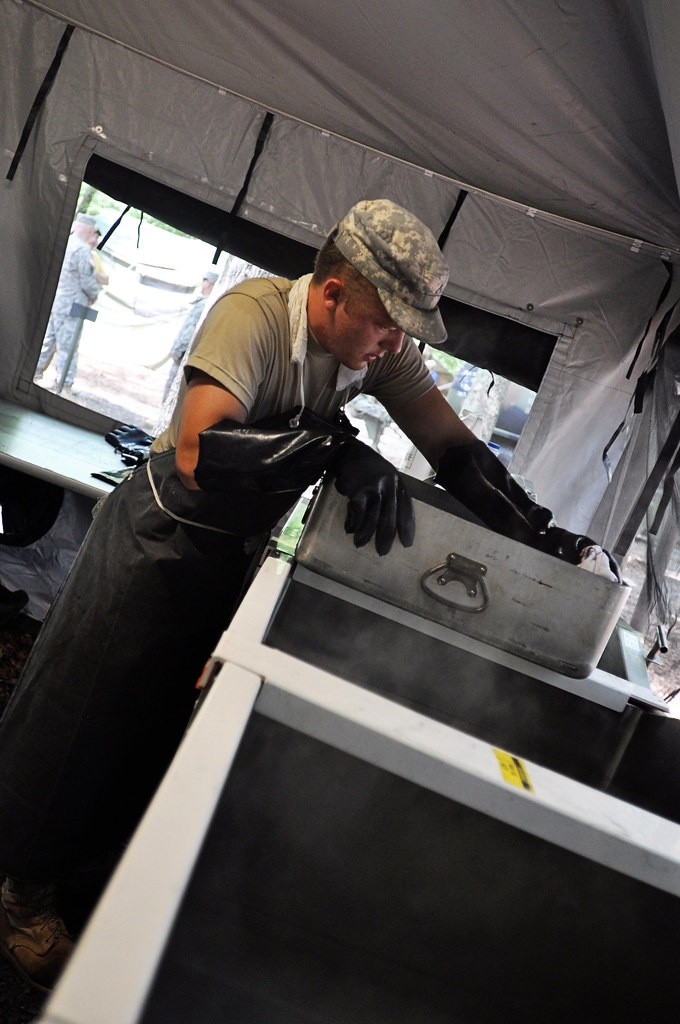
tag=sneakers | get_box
[1,882,72,993]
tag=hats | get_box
[332,198,450,343]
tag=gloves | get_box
[433,441,597,566]
[193,416,417,557]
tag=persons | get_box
[423,359,438,386]
[32,212,109,397]
[161,273,218,406]
[0,200,619,992]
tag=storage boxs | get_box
[286,438,632,681]
